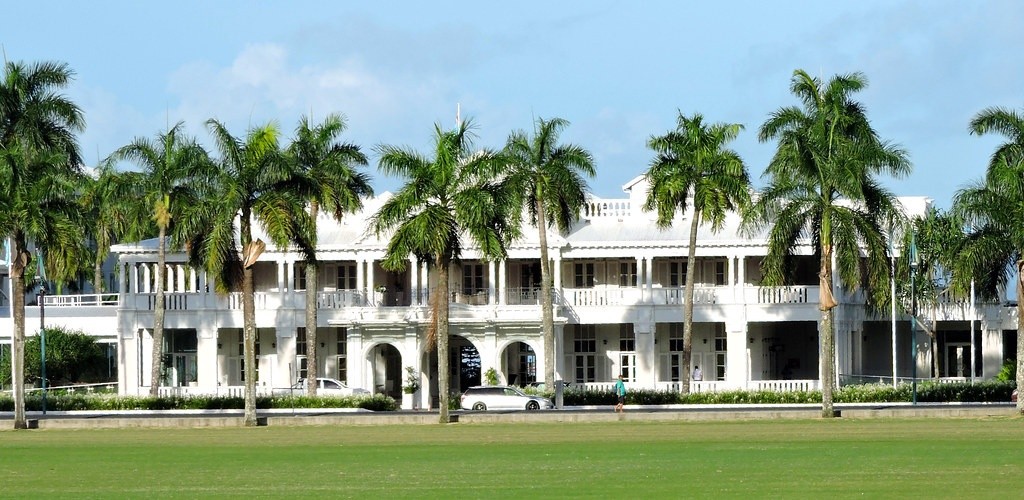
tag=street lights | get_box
[909,234,920,403]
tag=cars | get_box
[459,386,554,412]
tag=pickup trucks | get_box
[271,376,370,397]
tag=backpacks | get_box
[612,383,617,392]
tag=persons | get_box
[692,365,703,393]
[614,375,626,413]
